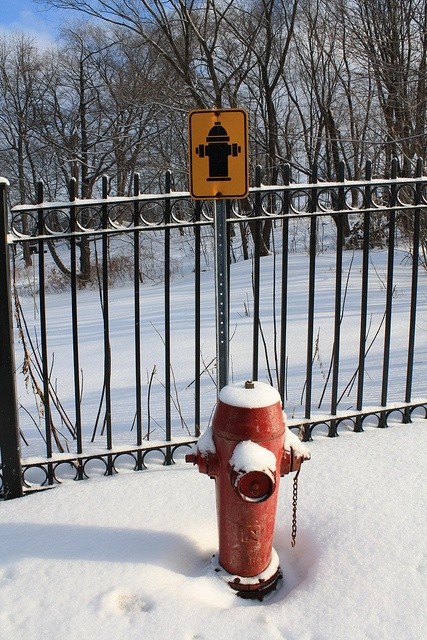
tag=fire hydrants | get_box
[183,380,311,603]
